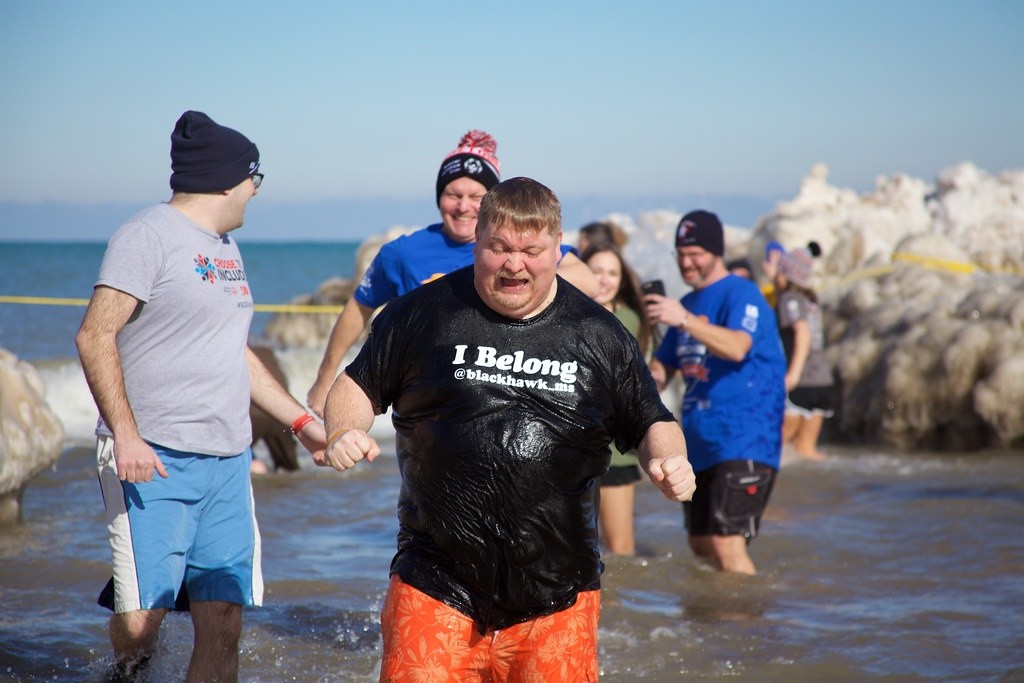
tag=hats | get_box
[437,130,500,207]
[167,110,260,193]
[676,210,725,257]
[779,241,822,288]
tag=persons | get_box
[308,131,601,422]
[74,111,330,683]
[774,241,841,467]
[645,207,790,574]
[726,260,752,283]
[324,177,698,682]
[578,223,626,265]
[581,242,666,558]
[758,241,792,323]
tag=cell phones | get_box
[642,280,665,306]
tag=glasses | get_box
[251,172,264,189]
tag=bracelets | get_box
[291,413,316,437]
[326,429,354,445]
[682,311,692,330]
